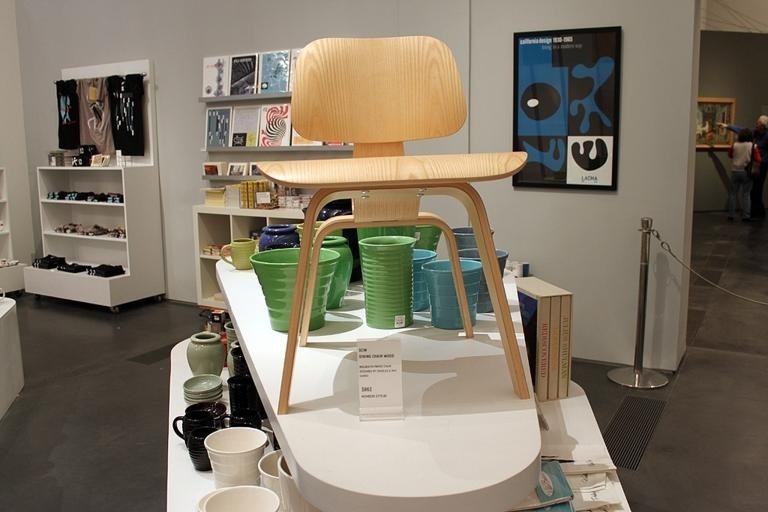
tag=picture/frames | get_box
[512,27,622,191]
[696,97,736,151]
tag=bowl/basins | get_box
[182,374,226,404]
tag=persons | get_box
[724,127,760,222]
[714,114,768,216]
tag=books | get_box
[201,48,343,258]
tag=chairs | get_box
[256,35,530,414]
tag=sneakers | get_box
[727,217,750,221]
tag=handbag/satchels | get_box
[745,161,759,177]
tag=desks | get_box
[166,256,633,512]
[1,297,25,422]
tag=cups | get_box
[220,222,508,334]
[172,401,320,512]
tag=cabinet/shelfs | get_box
[24,162,166,314]
[0,168,26,295]
[192,92,353,313]
